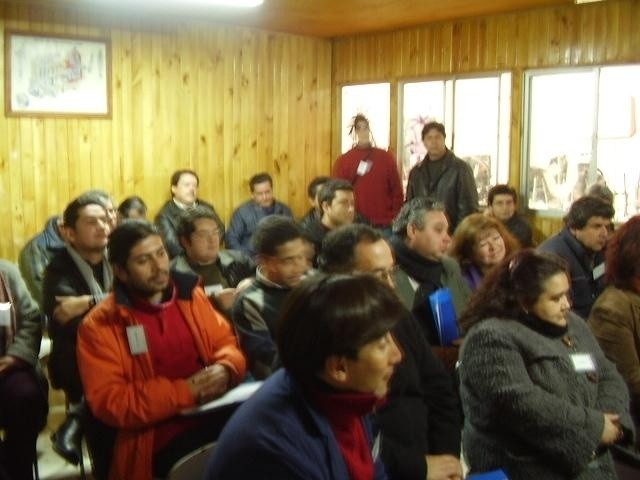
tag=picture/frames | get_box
[4,26,113,119]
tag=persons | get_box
[486,182,533,247]
[388,195,476,369]
[535,194,615,320]
[447,213,523,293]
[585,215,639,479]
[330,114,404,238]
[589,182,615,233]
[405,121,480,234]
[455,248,636,480]
[319,223,465,479]
[1,169,408,479]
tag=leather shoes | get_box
[49,411,89,467]
